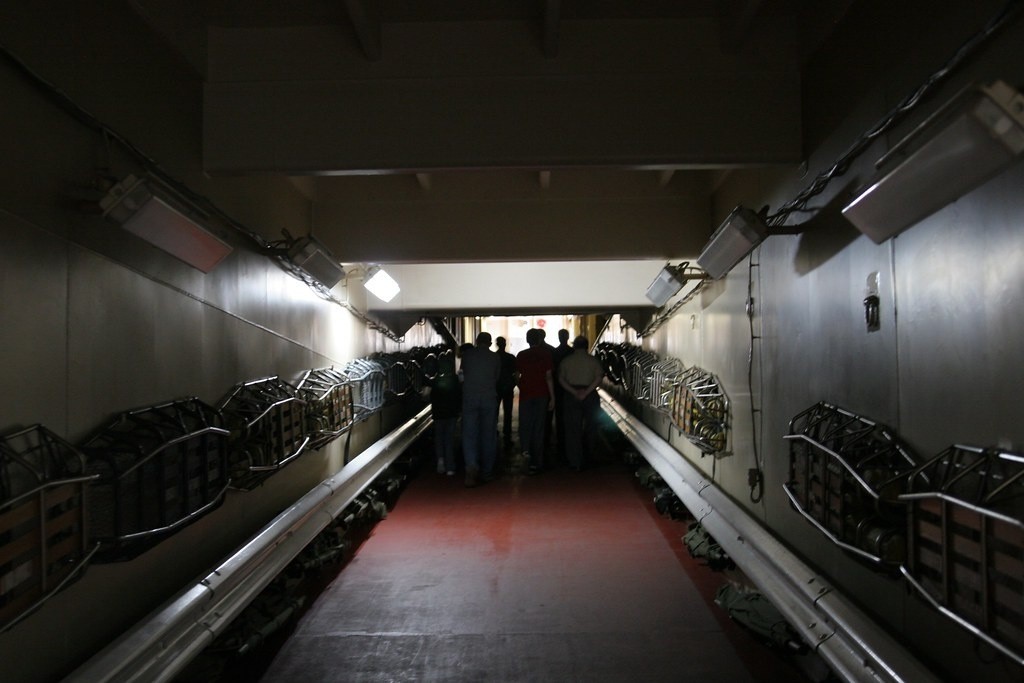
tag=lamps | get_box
[646,265,687,309]
[696,203,772,281]
[362,266,400,304]
[286,233,346,289]
[101,173,234,275]
[840,80,1024,245]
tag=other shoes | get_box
[528,468,541,475]
[521,448,530,460]
[571,463,584,471]
[465,480,477,489]
[480,477,490,484]
[445,468,457,476]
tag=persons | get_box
[559,336,606,472]
[429,328,573,489]
